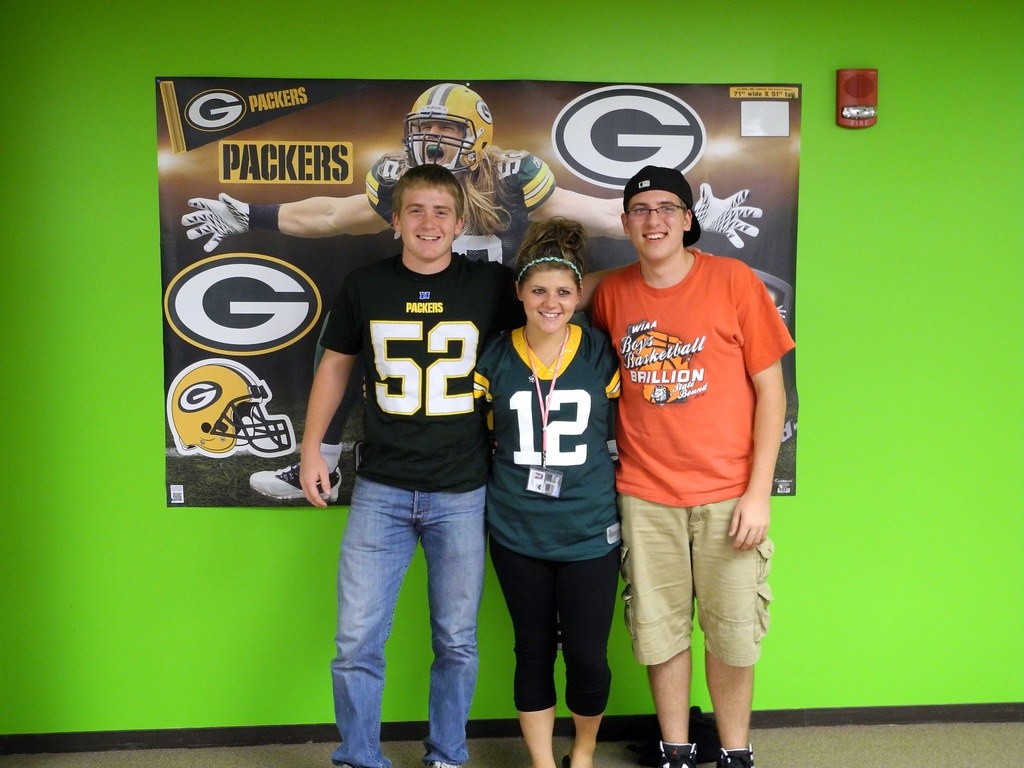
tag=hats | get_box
[622,164,702,249]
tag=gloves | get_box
[693,182,762,249]
[181,192,250,253]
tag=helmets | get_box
[400,83,493,176]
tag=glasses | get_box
[626,204,686,216]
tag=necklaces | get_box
[528,335,564,384]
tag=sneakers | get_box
[249,459,342,502]
[658,739,698,768]
[716,744,755,768]
[424,758,463,768]
[338,760,356,768]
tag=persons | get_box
[182,84,763,502]
[589,166,798,768]
[300,163,517,767]
[473,216,623,768]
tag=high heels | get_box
[560,755,571,768]
